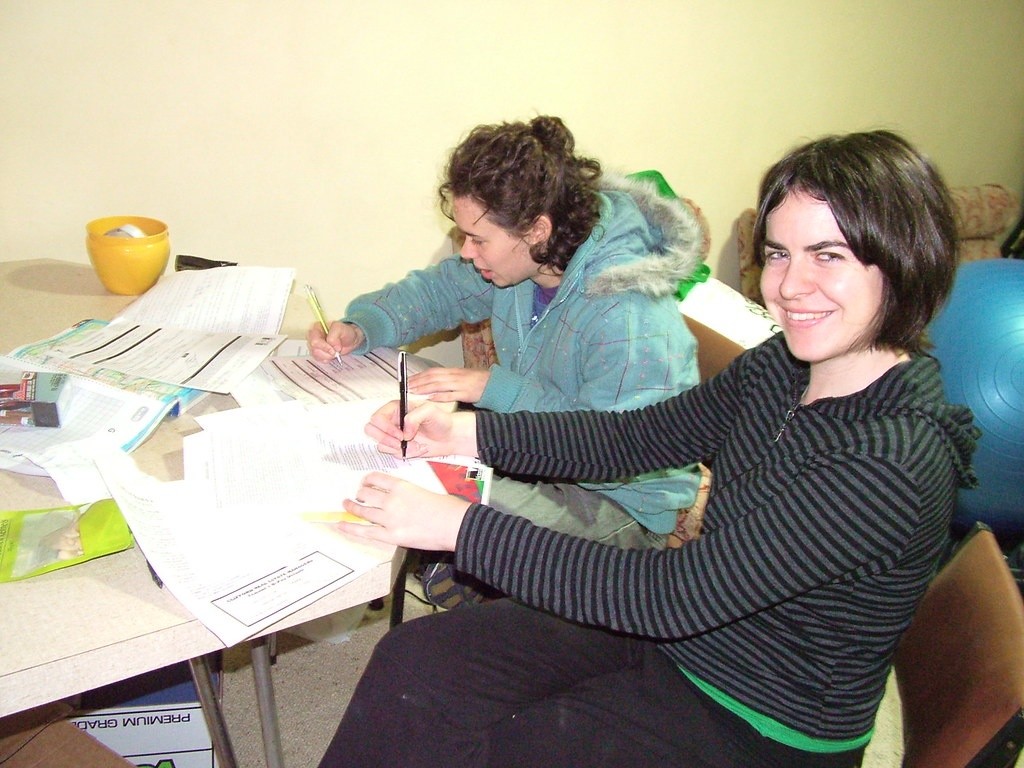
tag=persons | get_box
[307,117,701,613]
[316,128,982,768]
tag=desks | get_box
[0,255,476,768]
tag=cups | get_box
[86,216,171,294]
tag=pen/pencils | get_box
[301,282,345,367]
[395,349,412,466]
[0,379,37,426]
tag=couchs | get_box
[738,183,1013,307]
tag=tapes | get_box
[103,224,145,238]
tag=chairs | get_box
[388,312,748,634]
[894,521,1024,768]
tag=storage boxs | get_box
[0,701,137,768]
[59,647,224,768]
